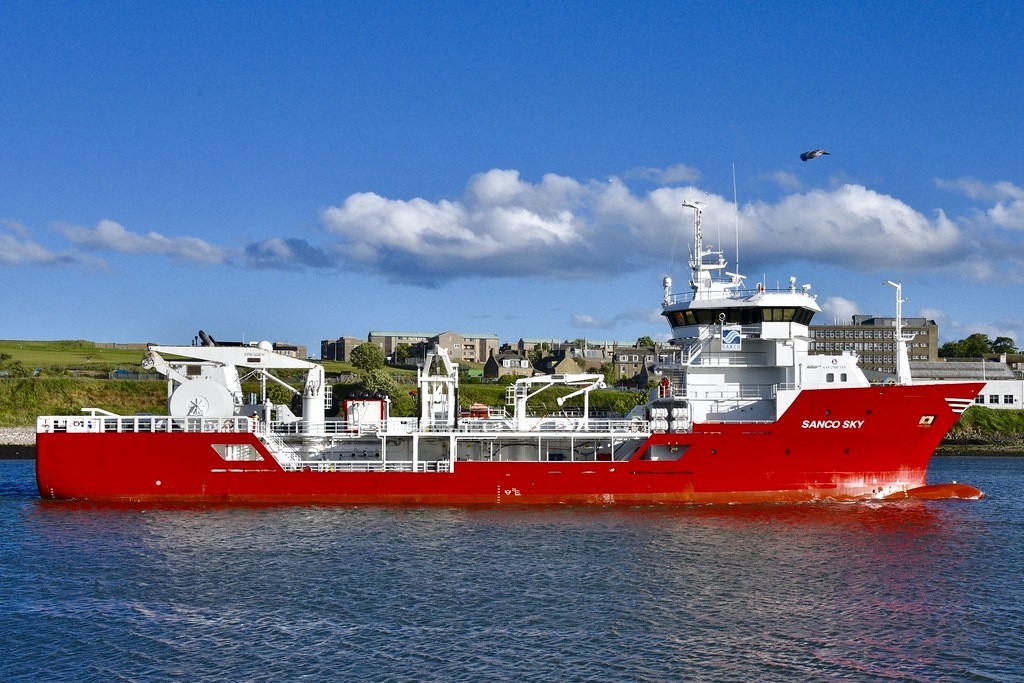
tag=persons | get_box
[657,376,670,398]
[251,411,259,420]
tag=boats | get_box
[36,160,988,507]
